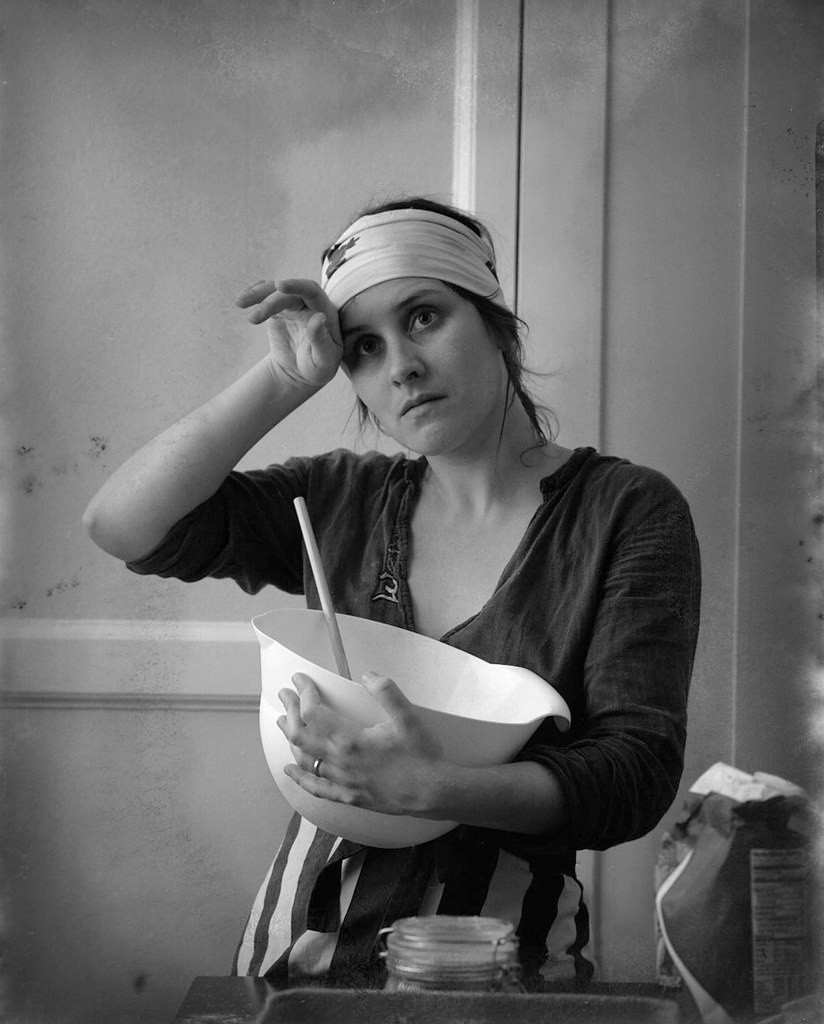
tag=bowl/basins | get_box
[251,606,572,850]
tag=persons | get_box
[81,190,703,993]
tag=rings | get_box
[313,758,323,776]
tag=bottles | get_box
[378,915,525,991]
